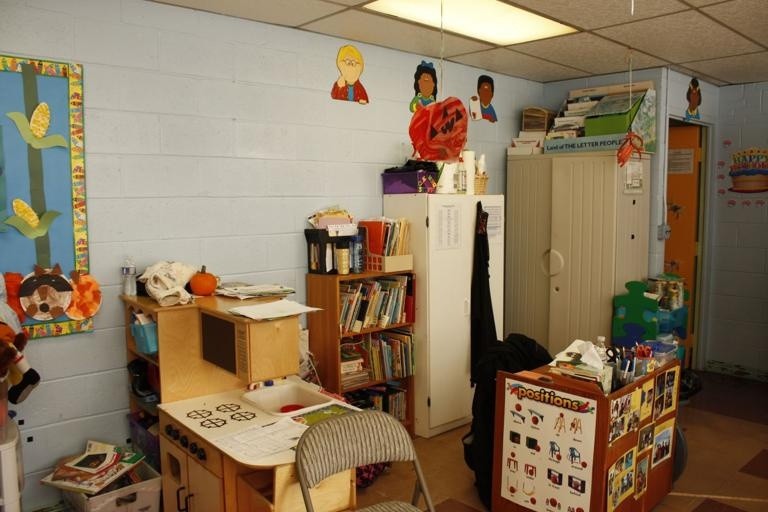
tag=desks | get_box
[490,351,681,511]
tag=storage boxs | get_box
[62,458,160,512]
[381,170,439,194]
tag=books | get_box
[340,327,416,385]
[338,274,413,333]
[345,384,408,424]
[360,217,411,273]
[39,440,148,496]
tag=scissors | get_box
[606,345,621,363]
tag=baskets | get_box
[474,175,490,195]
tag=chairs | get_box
[298,412,438,512]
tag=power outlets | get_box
[657,225,671,242]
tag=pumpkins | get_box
[190,266,216,296]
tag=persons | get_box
[330,44,370,105]
[680,78,704,121]
[466,74,499,124]
[408,60,439,114]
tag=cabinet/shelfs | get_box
[304,270,418,440]
[119,281,299,470]
[382,192,507,439]
[508,154,649,360]
[158,378,356,512]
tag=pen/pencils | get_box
[635,339,657,358]
[615,346,636,378]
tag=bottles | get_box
[121,256,137,296]
[477,153,485,174]
[593,335,607,364]
[335,239,349,275]
[456,157,467,194]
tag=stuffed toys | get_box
[0,322,42,406]
